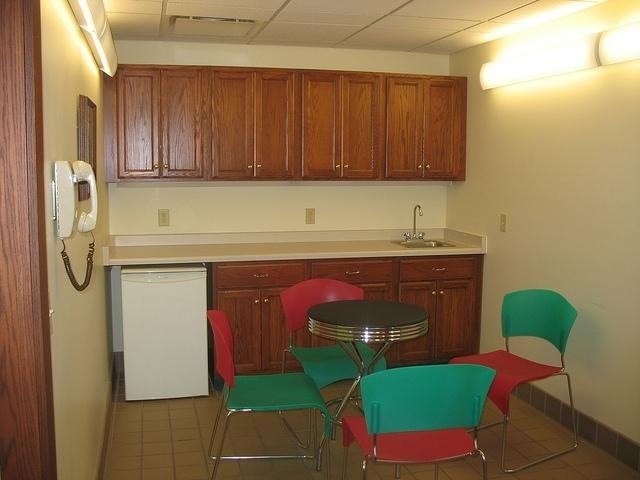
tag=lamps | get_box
[596,20,640,66]
[67,0,117,77]
[479,33,597,91]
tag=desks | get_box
[306,299,429,470]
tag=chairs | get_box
[339,363,497,480]
[206,310,333,480]
[276,278,387,450]
[445,290,577,473]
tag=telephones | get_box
[55,161,98,239]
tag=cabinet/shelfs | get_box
[211,254,484,390]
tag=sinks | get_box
[391,241,457,249]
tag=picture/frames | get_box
[77,94,97,201]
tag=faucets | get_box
[413,204,423,238]
